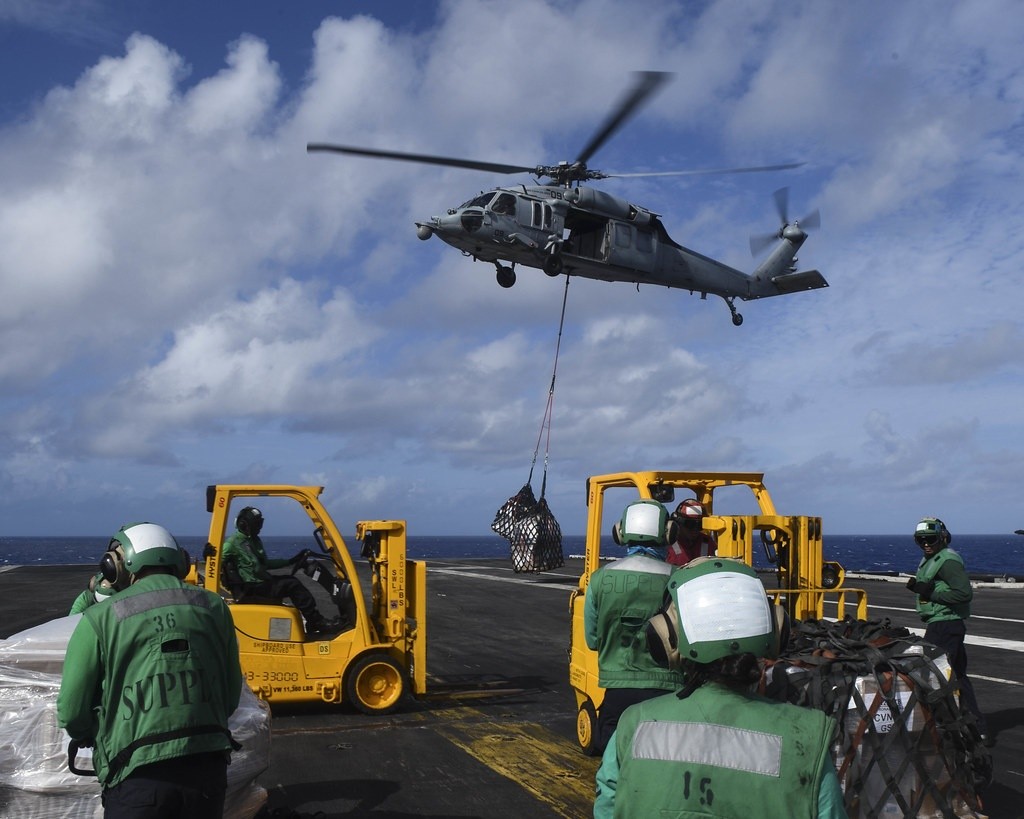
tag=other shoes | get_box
[305,618,337,634]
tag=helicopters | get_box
[304,72,830,327]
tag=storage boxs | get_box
[767,624,985,819]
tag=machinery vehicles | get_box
[183,484,546,716]
[566,470,873,756]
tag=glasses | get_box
[916,536,935,547]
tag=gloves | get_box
[913,582,932,598]
[288,548,307,565]
[907,577,917,591]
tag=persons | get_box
[666,499,718,566]
[220,507,347,633]
[584,500,680,755]
[593,555,847,819]
[56,522,242,819]
[905,516,985,722]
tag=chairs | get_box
[224,555,279,605]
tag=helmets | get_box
[109,523,191,583]
[235,507,264,536]
[914,518,952,554]
[645,556,790,664]
[613,500,669,547]
[676,499,708,520]
[92,572,118,603]
